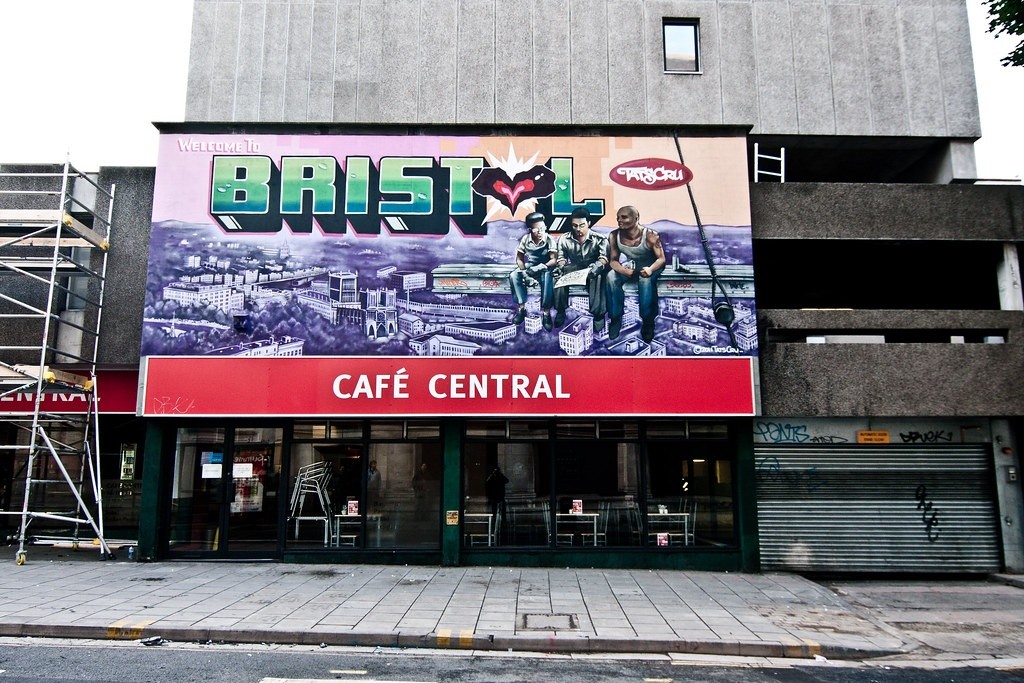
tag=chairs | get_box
[290,444,698,547]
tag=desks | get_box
[288,517,329,547]
[647,512,690,546]
[334,513,384,547]
[556,512,600,547]
[464,513,494,547]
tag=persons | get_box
[412,461,430,522]
[482,466,509,523]
[367,460,381,512]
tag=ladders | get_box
[753,141,787,183]
